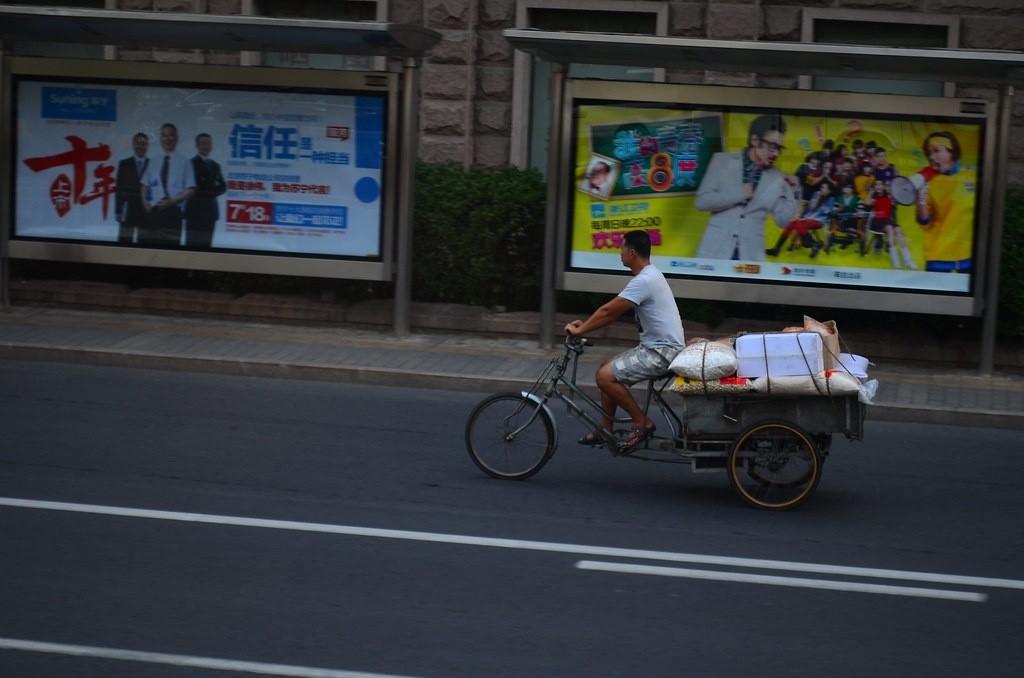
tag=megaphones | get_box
[889,166,939,205]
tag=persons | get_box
[114,123,228,248]
[580,161,613,197]
[563,230,685,449]
[693,113,978,275]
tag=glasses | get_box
[762,139,788,154]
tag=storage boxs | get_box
[822,320,842,368]
[735,332,824,377]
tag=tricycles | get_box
[465,328,867,512]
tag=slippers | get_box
[623,423,656,450]
[578,432,601,444]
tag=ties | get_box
[160,155,169,189]
[137,161,142,175]
[205,158,213,169]
[752,168,757,186]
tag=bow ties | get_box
[590,185,600,191]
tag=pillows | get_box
[835,353,870,378]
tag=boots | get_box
[802,234,821,258]
[901,248,918,269]
[889,248,900,269]
[767,233,786,256]
